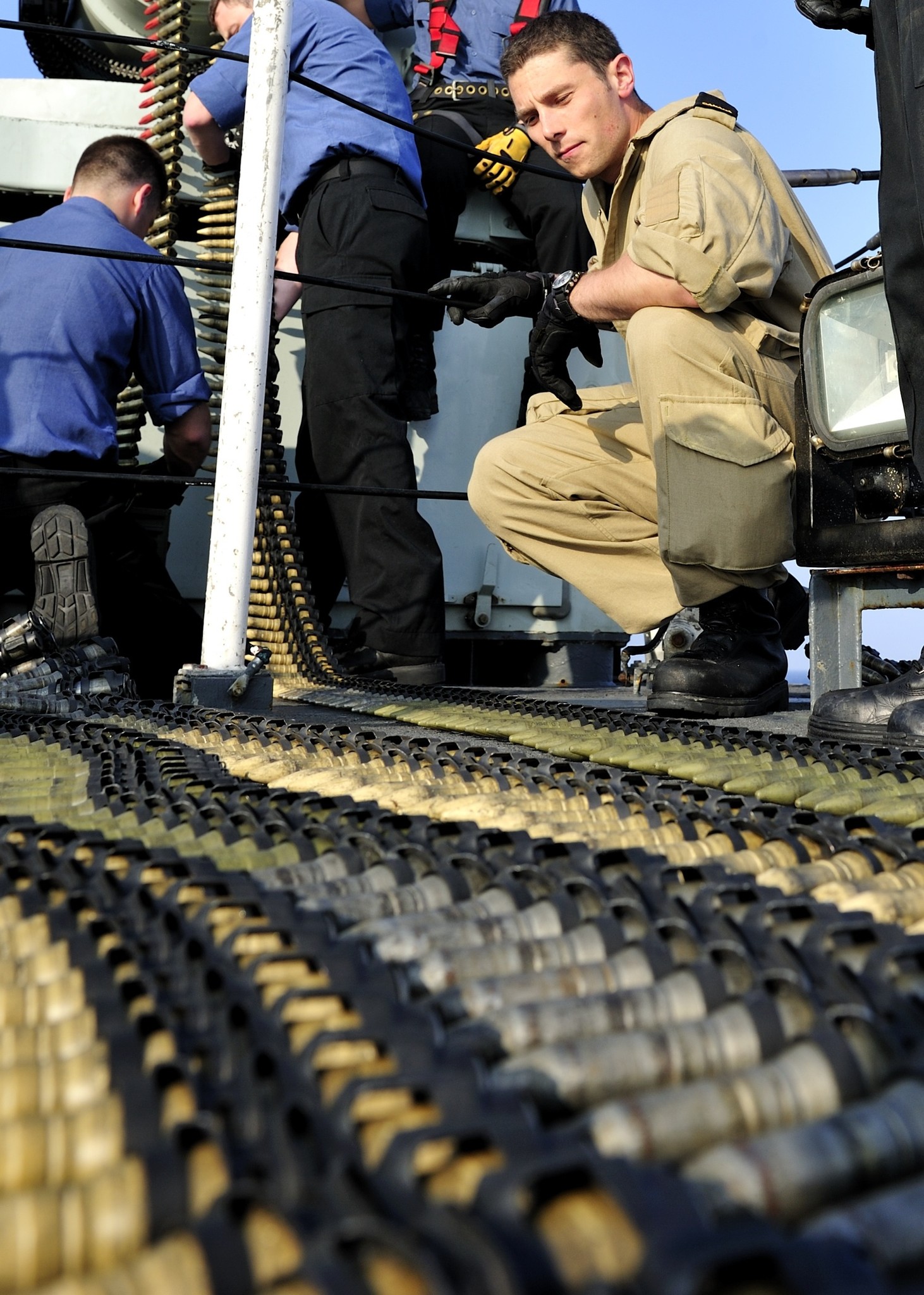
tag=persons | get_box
[2,135,218,674]
[792,1,923,757]
[421,8,850,716]
[329,0,595,422]
[183,1,459,680]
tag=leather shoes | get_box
[647,585,790,717]
[766,573,809,650]
[808,645,924,744]
[334,643,446,686]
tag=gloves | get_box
[428,270,554,329]
[529,294,603,411]
[467,122,532,196]
[202,147,240,181]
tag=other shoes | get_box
[27,504,97,650]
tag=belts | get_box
[311,160,395,193]
[408,79,512,101]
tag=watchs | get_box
[549,269,590,326]
